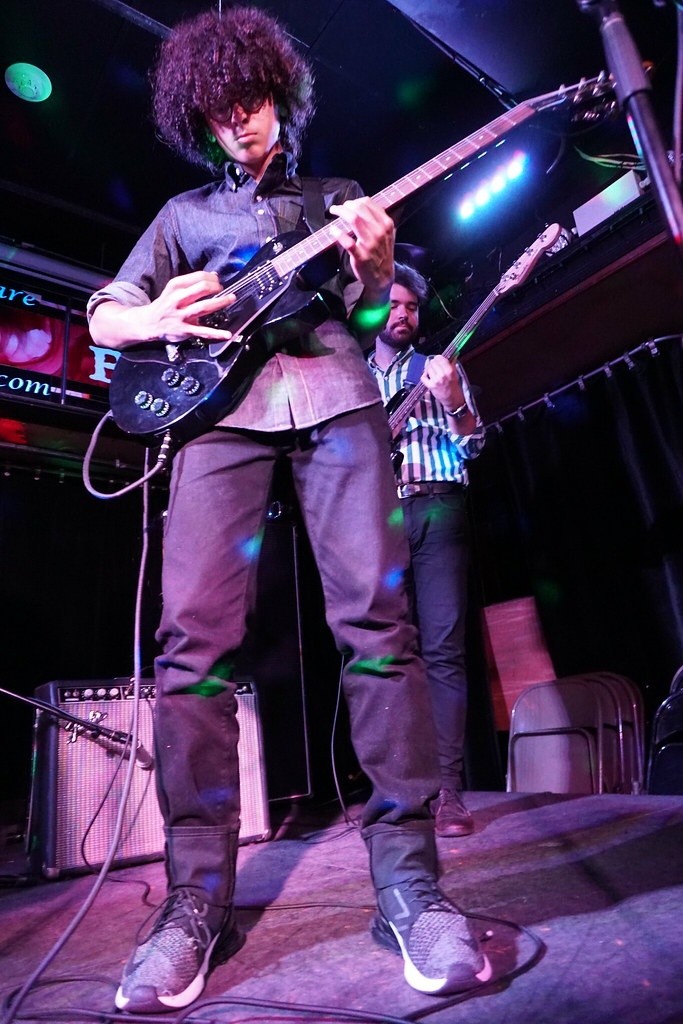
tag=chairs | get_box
[505,666,682,797]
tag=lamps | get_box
[5,62,53,104]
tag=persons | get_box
[87,6,492,1012]
[366,259,485,837]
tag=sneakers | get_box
[369,876,492,996]
[114,892,248,1013]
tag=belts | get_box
[395,482,462,499]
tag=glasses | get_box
[205,88,269,124]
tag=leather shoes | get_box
[429,785,476,837]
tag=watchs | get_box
[444,399,468,418]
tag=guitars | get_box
[383,220,569,475]
[110,54,652,452]
